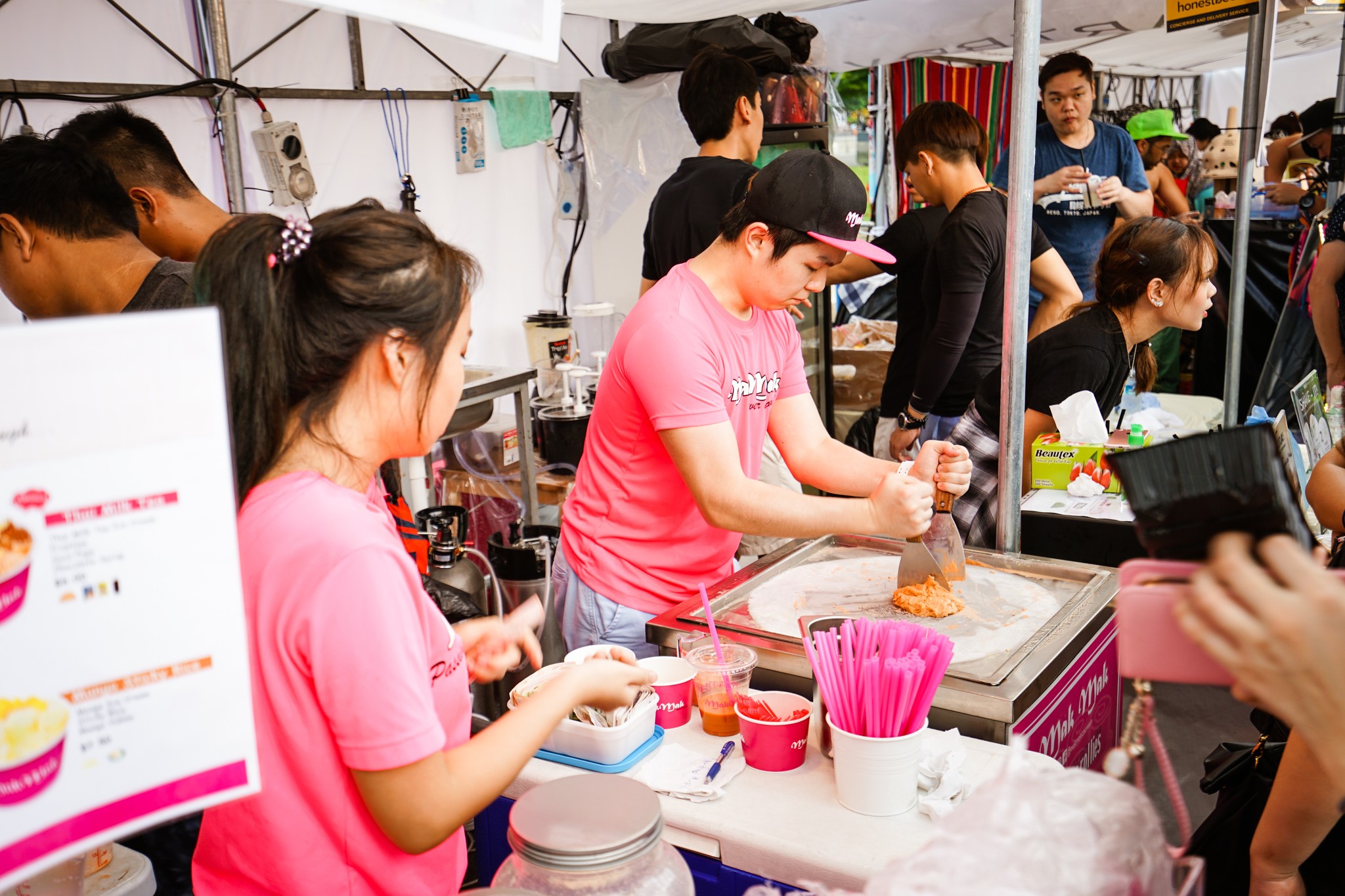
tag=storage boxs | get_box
[1031,429,1154,495]
[801,317,898,411]
[442,413,519,471]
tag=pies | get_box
[746,553,1058,664]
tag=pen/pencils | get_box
[705,741,735,785]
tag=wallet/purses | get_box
[1116,558,1345,684]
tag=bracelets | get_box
[902,406,928,423]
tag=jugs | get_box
[520,310,580,384]
[572,301,629,368]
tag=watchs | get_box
[896,413,926,430]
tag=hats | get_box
[1126,108,1191,141]
[744,147,897,264]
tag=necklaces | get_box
[1118,309,1138,379]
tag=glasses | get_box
[1146,139,1171,155]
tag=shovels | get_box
[922,453,965,583]
[898,482,950,592]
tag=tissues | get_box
[1031,390,1154,492]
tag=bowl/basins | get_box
[564,643,697,730]
[0,561,30,620]
[735,691,814,772]
[506,698,660,765]
[1,698,72,803]
[507,773,664,889]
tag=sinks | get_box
[442,368,493,437]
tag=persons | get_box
[553,146,974,664]
[891,100,1083,461]
[825,165,949,460]
[1166,96,1345,896]
[1112,108,1203,227]
[994,52,1154,310]
[175,196,657,896]
[638,52,812,320]
[0,107,241,896]
[945,211,1217,550]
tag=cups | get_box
[809,619,876,757]
[534,358,570,403]
[827,711,930,817]
[1082,175,1103,209]
[529,383,601,473]
[687,643,758,737]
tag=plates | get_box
[492,842,695,896]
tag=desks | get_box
[440,450,576,538]
